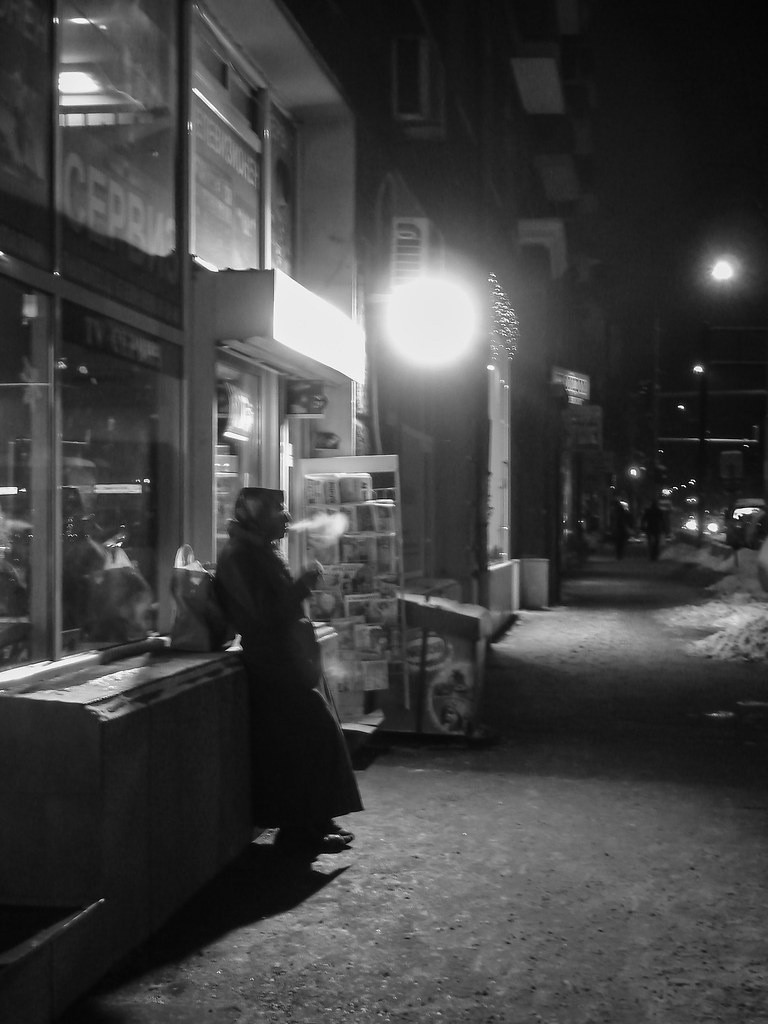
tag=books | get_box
[306,472,398,724]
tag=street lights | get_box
[694,252,743,535]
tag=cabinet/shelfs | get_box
[0,620,339,935]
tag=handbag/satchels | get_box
[169,544,236,653]
[103,548,152,643]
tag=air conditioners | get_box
[389,215,448,291]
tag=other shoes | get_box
[319,833,345,853]
[334,828,354,845]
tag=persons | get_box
[217,488,363,854]
[0,503,134,653]
[640,496,666,560]
[605,502,638,558]
